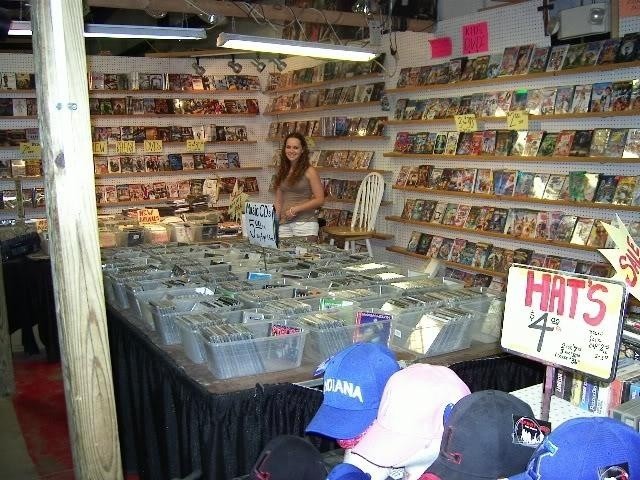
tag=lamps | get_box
[216,30,382,63]
[7,0,208,41]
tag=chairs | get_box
[322,169,385,259]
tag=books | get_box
[551,306,640,432]
[1,72,262,247]
[264,53,384,248]
[392,32,640,294]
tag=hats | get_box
[508,416,639,480]
[418,389,545,479]
[324,463,371,480]
[350,361,472,469]
[304,341,400,440]
[233,434,329,480]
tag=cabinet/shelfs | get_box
[261,69,393,239]
[2,87,264,232]
[386,60,640,307]
